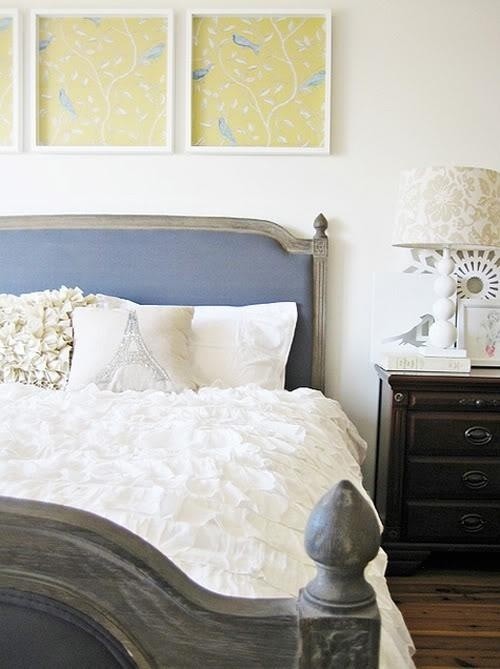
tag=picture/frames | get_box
[0,9,19,155]
[457,300,500,368]
[181,7,333,156]
[27,9,174,156]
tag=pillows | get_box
[65,306,200,396]
[0,284,99,392]
[83,293,298,392]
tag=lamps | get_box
[390,165,500,358]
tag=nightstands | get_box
[373,363,499,577]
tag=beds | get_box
[0,212,419,669]
